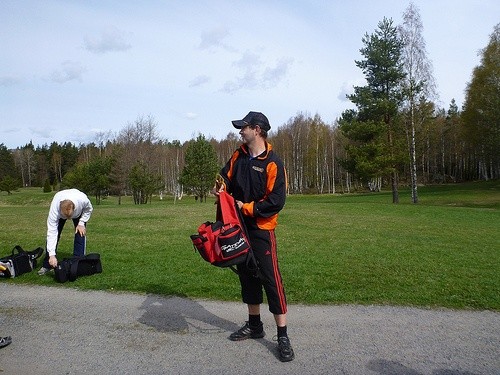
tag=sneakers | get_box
[37,267,54,276]
[230,320,266,341]
[272,335,295,362]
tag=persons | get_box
[37,188,94,277]
[213,111,295,362]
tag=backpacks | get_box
[189,190,260,280]
[0,245,44,279]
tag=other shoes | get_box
[0,335,13,348]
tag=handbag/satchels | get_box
[55,252,102,283]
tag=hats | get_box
[231,111,271,131]
